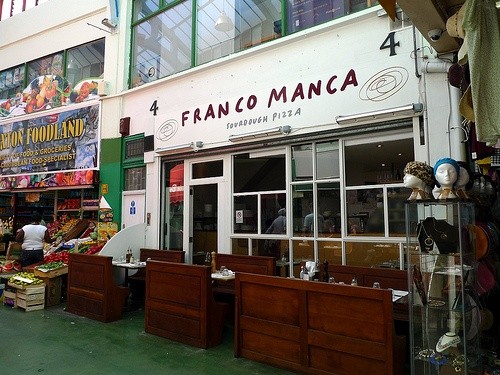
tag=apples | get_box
[83,245,99,255]
[38,261,64,270]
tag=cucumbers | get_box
[69,244,89,253]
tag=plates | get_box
[0,74,100,120]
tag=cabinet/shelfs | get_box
[0,194,71,220]
[405,196,482,375]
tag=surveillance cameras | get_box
[428,29,443,42]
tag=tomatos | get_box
[44,251,68,263]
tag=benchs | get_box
[64,248,419,375]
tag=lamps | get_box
[228,125,282,143]
[68,49,80,75]
[337,104,414,126]
[156,141,196,154]
[215,0,234,32]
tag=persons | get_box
[32,210,46,225]
[403,158,474,200]
[322,211,336,233]
[263,207,285,254]
[302,204,324,233]
[15,212,51,267]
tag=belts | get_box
[24,247,42,250]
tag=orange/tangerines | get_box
[62,218,78,233]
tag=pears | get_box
[9,271,44,285]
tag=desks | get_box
[391,283,408,302]
[210,271,235,281]
[112,261,147,311]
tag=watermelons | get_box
[0,262,23,273]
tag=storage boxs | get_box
[0,215,104,311]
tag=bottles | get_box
[125,247,134,263]
[312,258,335,283]
[204,251,216,274]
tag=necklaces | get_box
[416,217,455,246]
[437,332,461,350]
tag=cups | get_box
[219,266,229,277]
[373,282,381,288]
[350,279,357,286]
[119,256,125,263]
